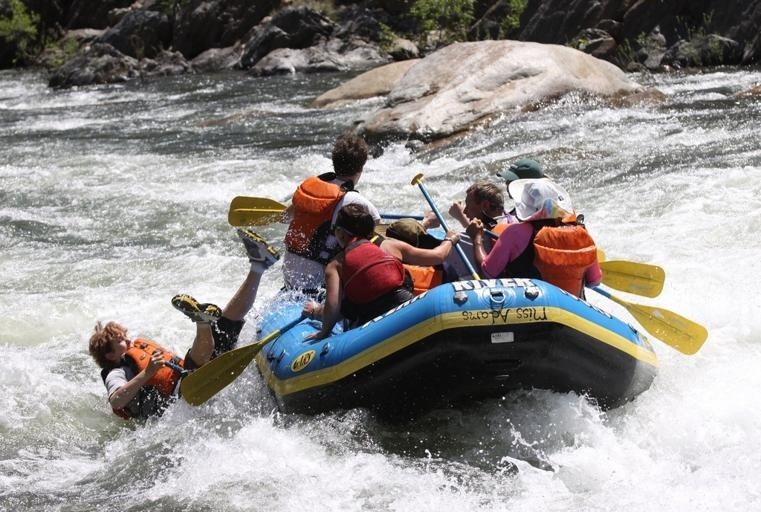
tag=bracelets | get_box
[444,237,455,245]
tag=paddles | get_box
[181,299,325,406]
[590,286,708,356]
[228,196,425,227]
[482,228,665,298]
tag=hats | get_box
[508,178,574,222]
[496,160,544,181]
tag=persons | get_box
[439,183,506,273]
[493,159,546,235]
[465,178,602,300]
[303,218,434,350]
[282,136,441,292]
[301,203,461,343]
[90,226,279,423]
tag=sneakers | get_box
[237,228,279,268]
[171,293,222,322]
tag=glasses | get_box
[331,223,353,236]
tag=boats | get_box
[246,281,657,416]
